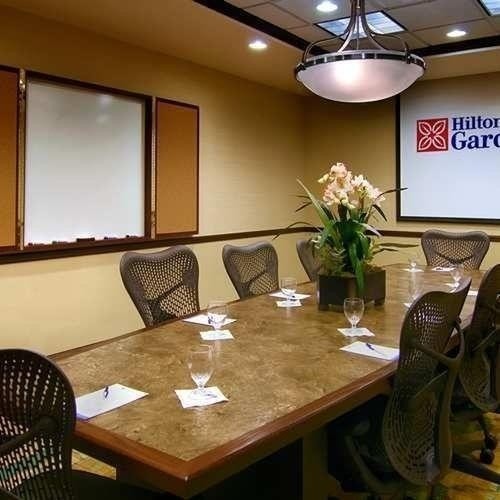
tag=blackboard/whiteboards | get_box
[15,67,157,254]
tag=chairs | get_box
[295,238,322,284]
[119,242,201,330]
[322,277,479,499]
[0,349,143,499]
[451,264,499,484]
[219,242,281,303]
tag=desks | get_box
[0,262,490,498]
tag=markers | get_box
[104,236,117,240]
[52,240,67,244]
[28,242,44,246]
[126,235,139,238]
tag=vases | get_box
[315,266,391,315]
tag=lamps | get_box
[289,0,432,107]
[416,229,494,269]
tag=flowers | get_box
[270,160,410,289]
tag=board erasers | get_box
[77,237,95,242]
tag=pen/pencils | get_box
[104,386,108,400]
[366,343,380,354]
